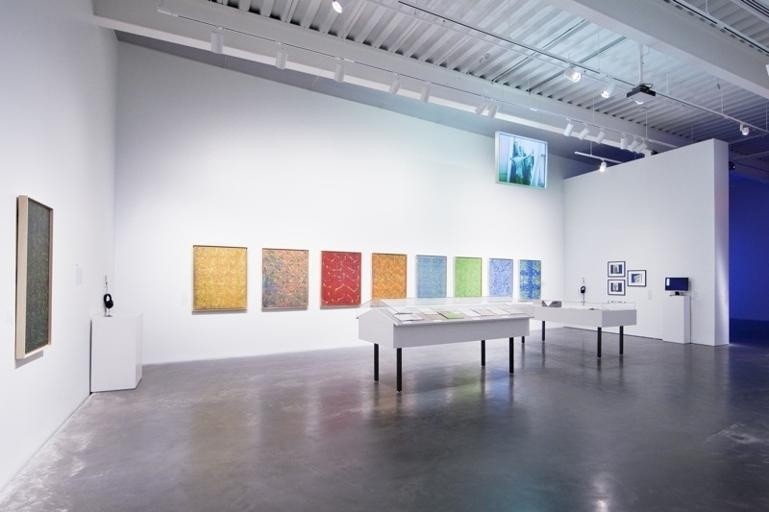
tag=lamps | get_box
[418,80,436,103]
[205,25,228,53]
[331,58,349,85]
[619,134,648,155]
[597,161,608,171]
[562,118,573,138]
[474,95,487,116]
[593,128,606,144]
[485,101,499,119]
[386,73,402,98]
[577,123,590,140]
[272,45,291,71]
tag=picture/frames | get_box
[607,279,625,295]
[517,258,541,303]
[191,244,250,315]
[413,252,449,299]
[487,256,515,303]
[607,261,624,277]
[16,194,53,362]
[370,251,409,301]
[260,246,312,312]
[452,255,484,300]
[320,250,364,310]
[627,270,646,286]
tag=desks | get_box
[522,305,637,358]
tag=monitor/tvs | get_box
[665,277,689,296]
[495,131,548,190]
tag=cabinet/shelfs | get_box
[354,297,536,393]
[91,312,142,392]
